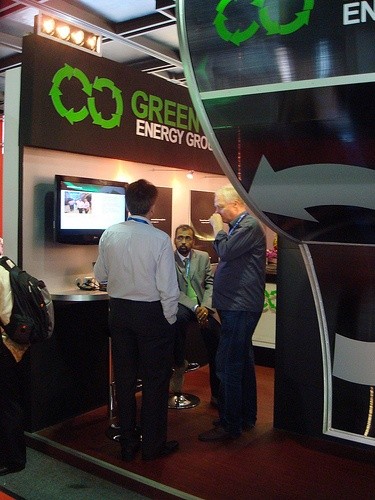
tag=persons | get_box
[197,184,268,441]
[173,224,222,408]
[0,238,30,476]
[93,178,180,462]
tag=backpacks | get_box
[0,255,56,346]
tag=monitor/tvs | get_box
[54,175,130,245]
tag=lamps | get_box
[184,169,194,179]
[35,11,102,57]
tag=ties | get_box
[183,257,193,281]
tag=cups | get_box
[84,277,95,289]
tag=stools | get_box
[107,297,145,445]
[168,322,205,410]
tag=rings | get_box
[204,313,207,315]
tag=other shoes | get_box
[141,439,182,462]
[197,423,242,442]
[210,420,226,427]
[0,464,13,477]
[122,443,143,461]
